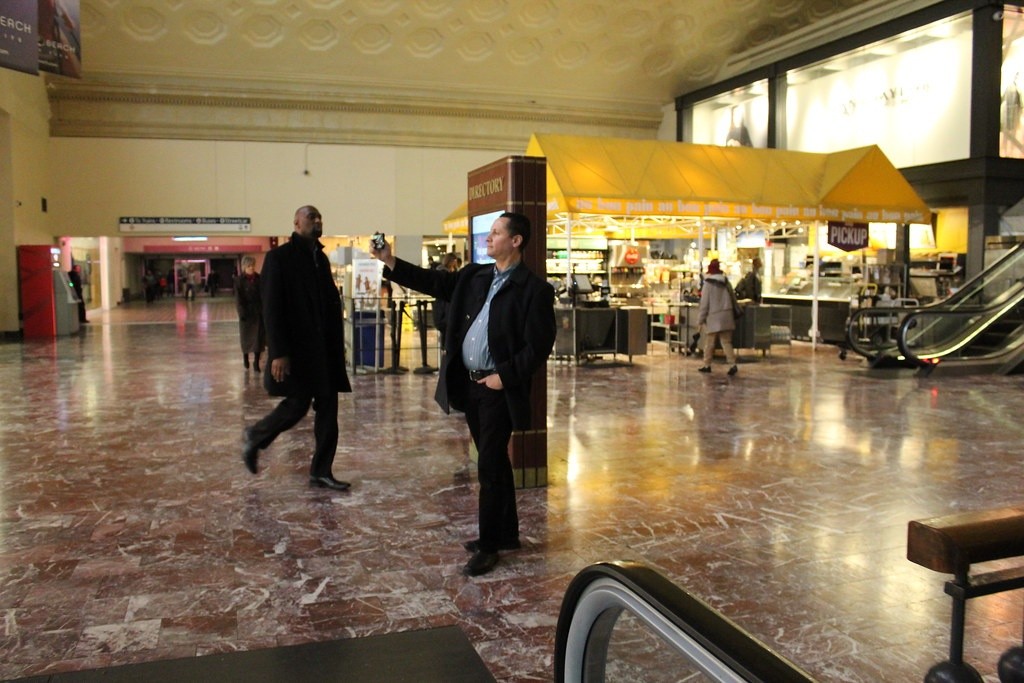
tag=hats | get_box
[706,258,724,275]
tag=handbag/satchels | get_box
[725,278,745,318]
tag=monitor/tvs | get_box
[571,274,593,294]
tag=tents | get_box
[442,134,931,353]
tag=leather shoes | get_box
[464,550,501,577]
[464,535,522,552]
[241,427,260,474]
[308,473,352,491]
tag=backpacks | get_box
[735,277,748,299]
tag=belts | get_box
[461,368,498,382]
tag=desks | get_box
[554,307,648,365]
[677,304,772,361]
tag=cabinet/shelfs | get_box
[650,302,689,359]
[547,237,650,300]
[859,263,909,308]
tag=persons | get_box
[233,256,265,372]
[185,265,196,301]
[68,265,89,323]
[369,213,557,576]
[242,206,351,490]
[734,272,763,304]
[436,253,462,271]
[753,254,763,281]
[428,257,438,269]
[698,259,737,375]
[143,270,158,305]
[208,269,218,297]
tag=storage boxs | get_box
[984,235,1024,249]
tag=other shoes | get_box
[243,353,250,369]
[728,365,739,378]
[252,360,261,373]
[698,367,712,374]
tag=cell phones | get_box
[371,234,385,250]
